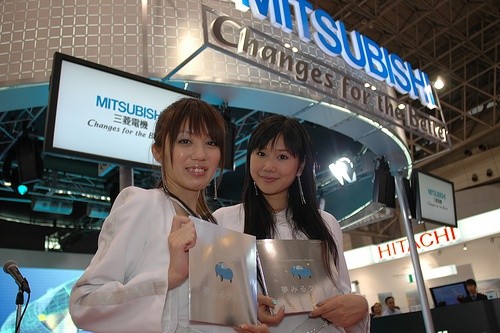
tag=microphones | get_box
[3,260,31,293]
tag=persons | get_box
[370,296,402,318]
[463,279,488,305]
[69,97,271,333]
[208,115,371,333]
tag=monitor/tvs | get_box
[43,51,200,172]
[412,168,457,227]
[372,170,410,208]
[429,282,470,308]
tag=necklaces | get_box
[164,187,199,218]
